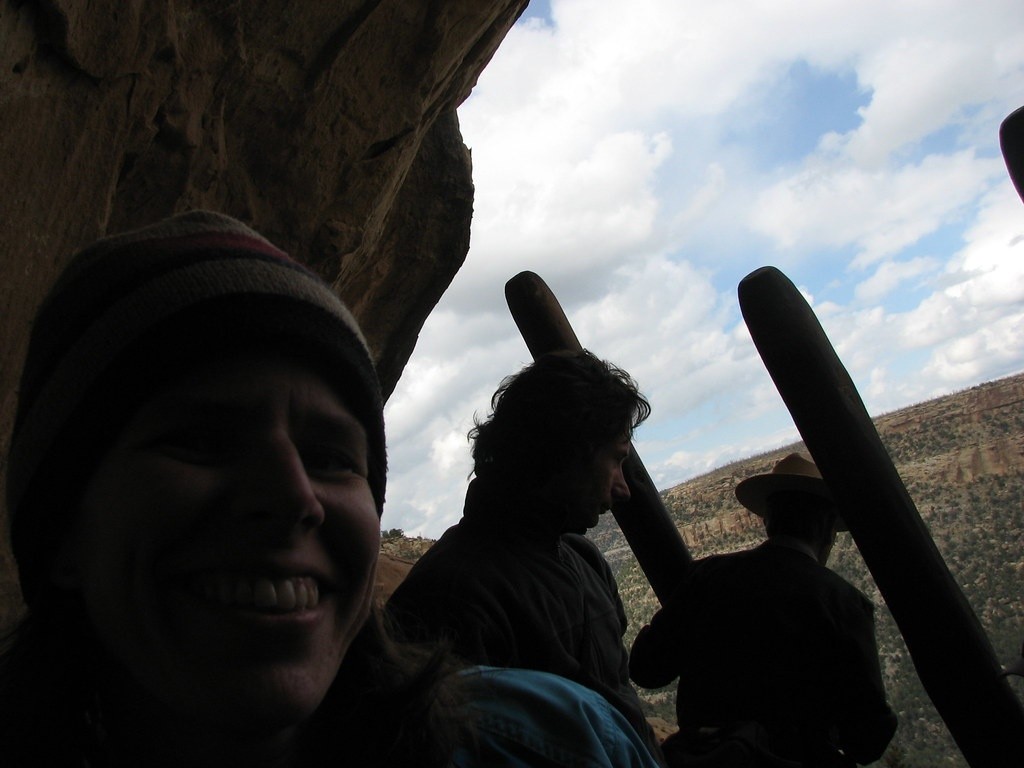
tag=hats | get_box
[736,454,847,532]
[8,210,384,600]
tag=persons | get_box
[388,349,665,768]
[627,453,898,768]
[1,208,665,768]
